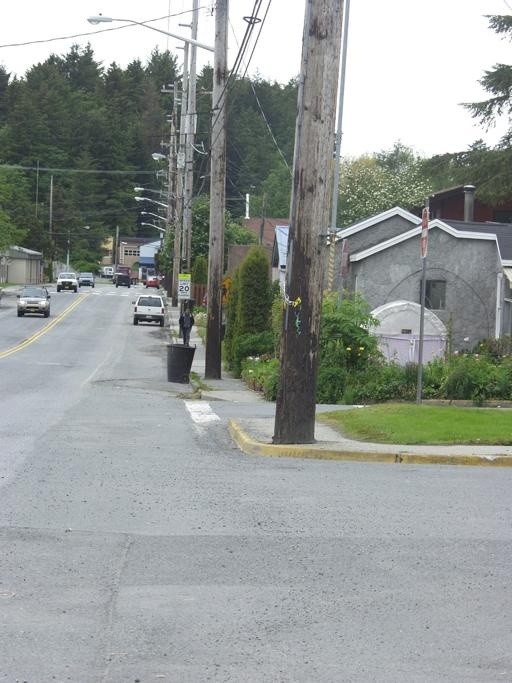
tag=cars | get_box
[17,265,95,326]
[131,294,165,326]
[99,264,160,289]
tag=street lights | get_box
[67,225,90,272]
[87,0,228,380]
[132,152,172,233]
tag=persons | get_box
[179,307,195,346]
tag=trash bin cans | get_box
[167,343,196,384]
